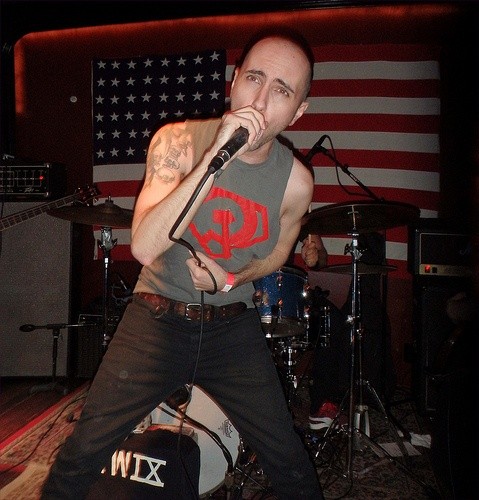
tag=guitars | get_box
[0,183,101,231]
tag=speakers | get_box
[413,278,474,415]
[0,198,83,379]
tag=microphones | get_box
[208,125,250,173]
[167,385,190,406]
[305,135,326,161]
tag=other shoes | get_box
[309,402,345,430]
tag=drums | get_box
[261,305,330,389]
[252,266,310,337]
[133,384,244,499]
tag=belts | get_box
[139,292,249,325]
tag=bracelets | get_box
[220,272,235,292]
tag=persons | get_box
[40,30,322,500]
[281,214,349,431]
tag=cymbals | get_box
[298,200,421,235]
[45,202,134,228]
[320,263,398,274]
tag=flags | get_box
[91,42,440,282]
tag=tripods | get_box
[310,148,413,477]
